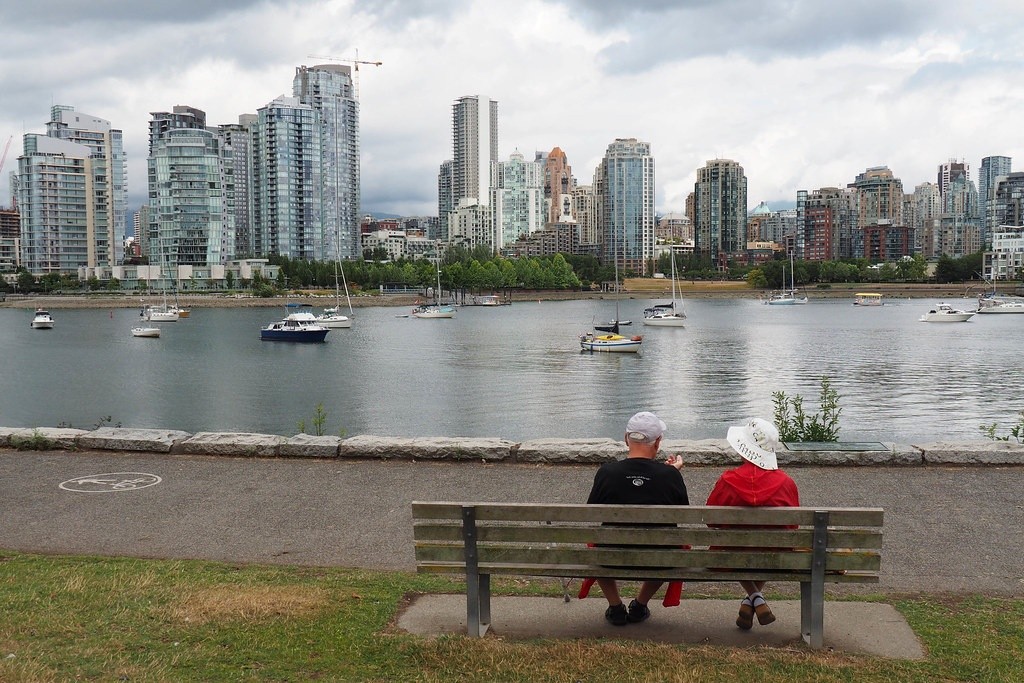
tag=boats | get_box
[918,303,977,322]
[972,265,1024,314]
[259,312,332,343]
[131,327,161,338]
[30,308,55,329]
[850,292,884,306]
[608,320,632,326]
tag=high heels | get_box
[736,597,754,630]
[752,596,776,626]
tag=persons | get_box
[578,411,691,625]
[702,417,801,631]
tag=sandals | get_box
[605,604,628,626]
[628,600,650,624]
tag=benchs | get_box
[411,500,885,650]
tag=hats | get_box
[626,412,667,442]
[726,418,779,470]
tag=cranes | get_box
[306,46,383,101]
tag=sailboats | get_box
[765,249,810,305]
[643,213,688,327]
[315,242,355,328]
[577,214,645,354]
[412,245,458,319]
[139,235,191,323]
